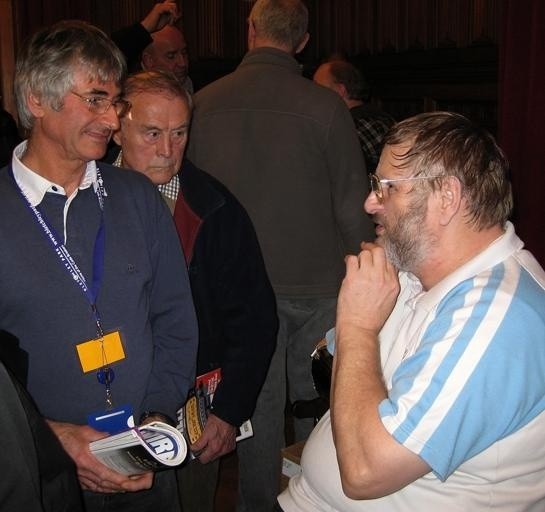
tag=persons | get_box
[1,0,398,511]
[274,111,545,511]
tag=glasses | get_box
[67,89,131,116]
[369,173,447,199]
